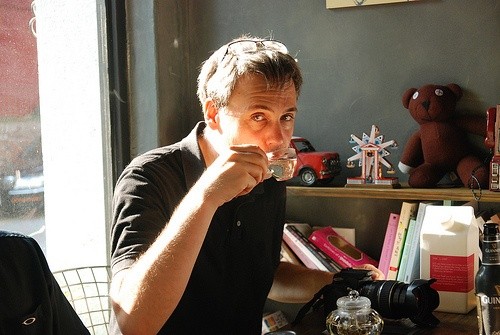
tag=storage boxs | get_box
[262,311,288,335]
[308,223,379,269]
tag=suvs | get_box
[285,136,342,186]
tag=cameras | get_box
[322,267,441,327]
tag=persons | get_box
[107,29,384,335]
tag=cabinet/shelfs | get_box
[256,186,500,335]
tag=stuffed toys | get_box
[398,83,489,189]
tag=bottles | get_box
[326,290,385,335]
[475,222,500,335]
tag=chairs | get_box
[49,266,113,335]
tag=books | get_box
[453,201,500,262]
[280,200,443,284]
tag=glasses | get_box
[205,40,288,98]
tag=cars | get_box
[8,169,45,212]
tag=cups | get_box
[265,147,298,180]
